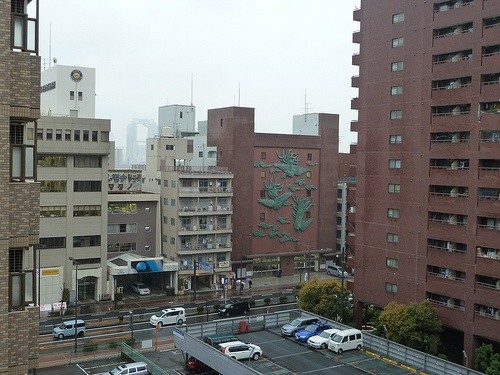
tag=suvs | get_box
[150,307,187,328]
[215,340,263,361]
[327,264,349,278]
[52,319,86,339]
[217,300,251,318]
[130,282,151,296]
[280,315,320,337]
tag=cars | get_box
[307,328,343,350]
[187,356,214,374]
[294,322,333,343]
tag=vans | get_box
[328,328,364,354]
[103,362,150,375]
[203,333,242,348]
